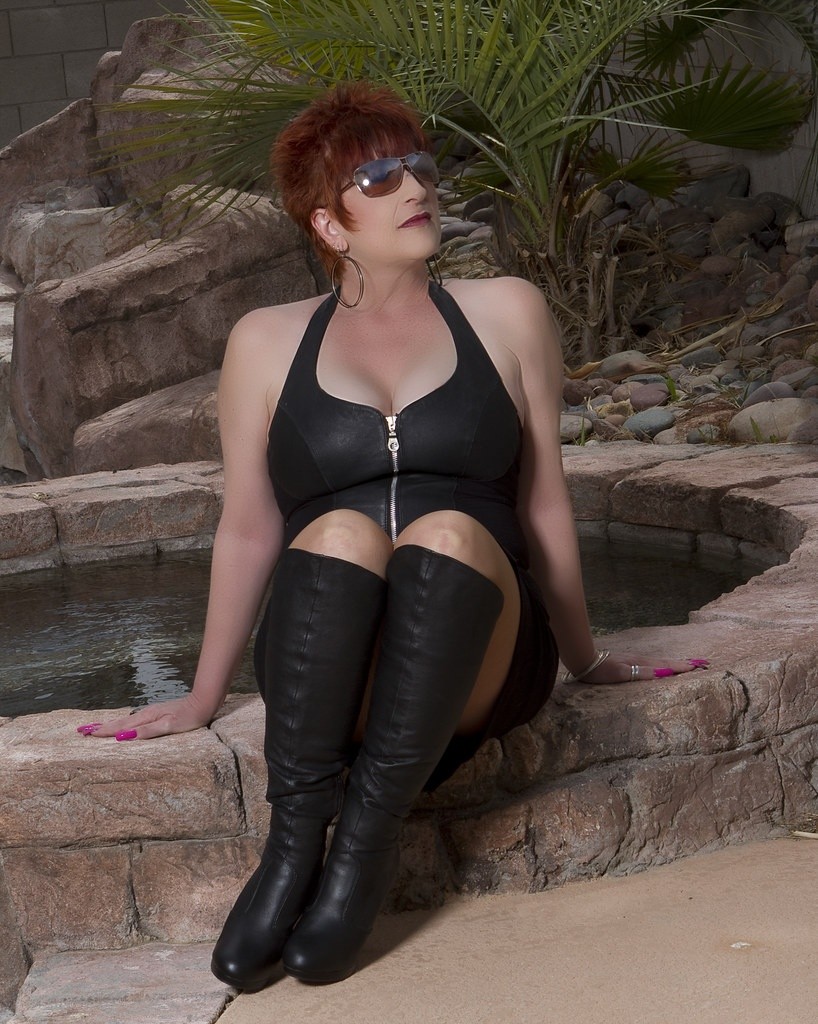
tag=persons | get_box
[77,80,713,994]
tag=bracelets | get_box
[560,648,610,685]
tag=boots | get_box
[210,549,387,994]
[282,544,506,985]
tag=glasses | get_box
[325,150,440,207]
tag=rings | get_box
[631,664,640,682]
